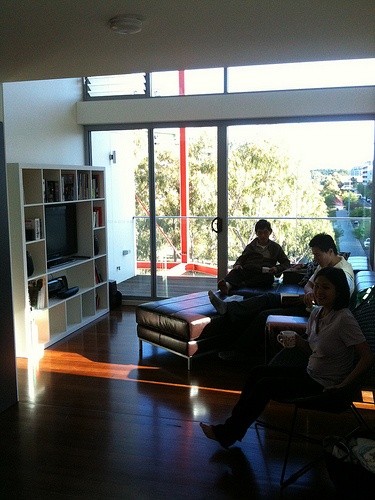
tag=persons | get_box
[198,266,370,449]
[217,220,292,296]
[207,232,355,362]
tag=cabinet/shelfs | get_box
[6,163,110,358]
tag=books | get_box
[25,215,41,242]
[95,263,104,284]
[95,288,100,311]
[93,206,103,228]
[42,171,105,203]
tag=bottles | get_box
[305,301,315,318]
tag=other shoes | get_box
[199,421,215,440]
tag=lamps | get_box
[109,150,116,164]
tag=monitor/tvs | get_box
[45,201,78,268]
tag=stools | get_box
[133,289,244,371]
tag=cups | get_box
[277,331,296,348]
[262,267,270,273]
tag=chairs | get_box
[255,385,375,496]
[262,269,375,415]
